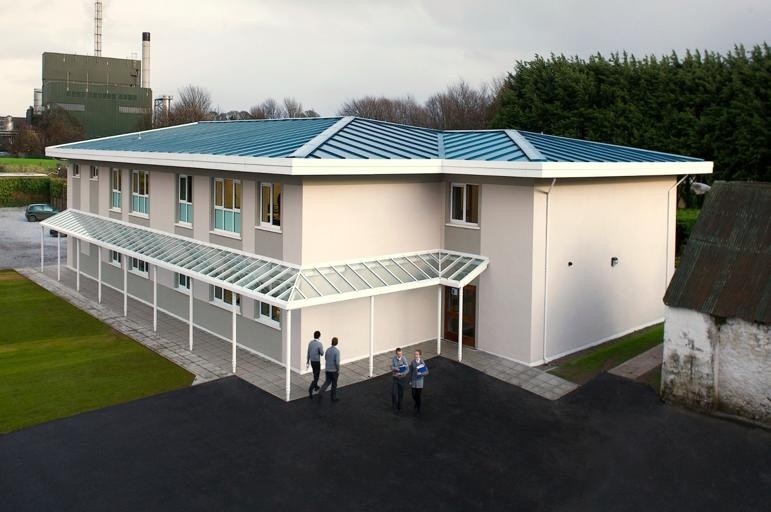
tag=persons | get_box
[390,348,410,415]
[306,331,325,400]
[317,337,343,402]
[410,349,430,417]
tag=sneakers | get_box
[332,398,341,401]
[392,405,403,416]
[314,386,321,391]
[414,405,422,418]
[309,389,314,400]
[318,390,324,399]
